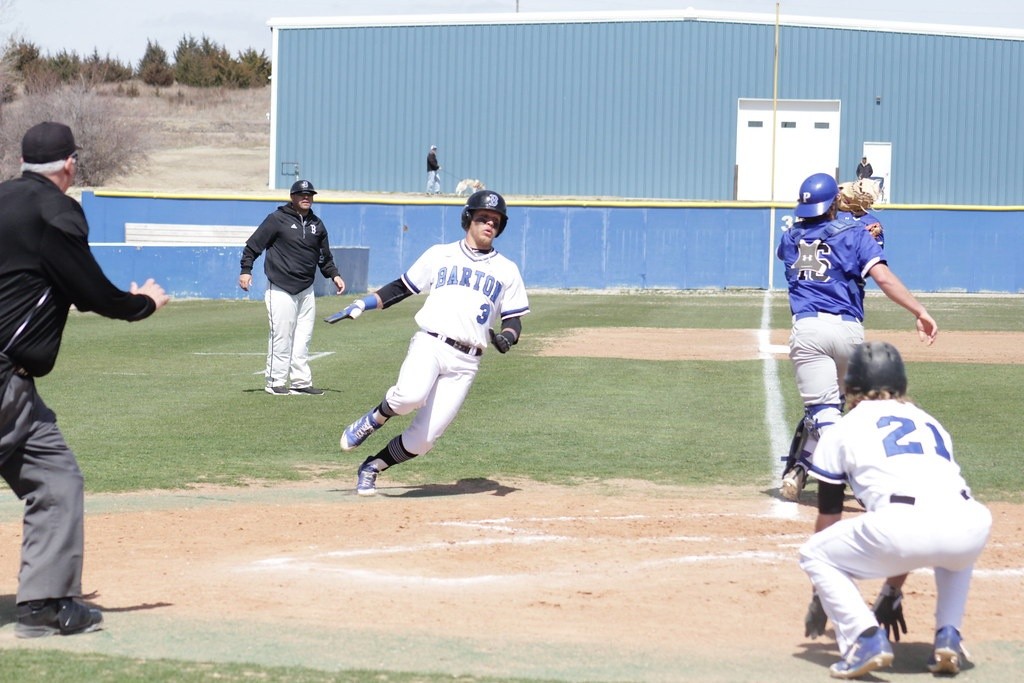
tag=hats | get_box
[23,122,83,163]
[431,145,437,151]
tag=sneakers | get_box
[265,386,291,395]
[14,598,102,640]
[925,625,961,674]
[357,456,380,496]
[339,407,383,451]
[829,628,894,678]
[289,387,324,396]
[781,466,806,501]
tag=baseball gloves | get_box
[865,222,883,238]
[838,179,881,212]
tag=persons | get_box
[1,121,170,638]
[798,342,993,679]
[239,180,345,395]
[427,145,442,194]
[777,172,938,499]
[837,209,884,249]
[856,157,873,179]
[324,191,530,496]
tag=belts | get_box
[890,491,970,504]
[427,332,483,356]
[796,312,858,322]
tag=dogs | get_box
[456,179,487,197]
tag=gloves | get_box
[805,595,828,639]
[324,300,365,324]
[489,328,511,354]
[872,583,908,641]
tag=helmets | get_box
[461,190,508,237]
[289,180,317,197]
[794,173,838,217]
[842,342,906,395]
